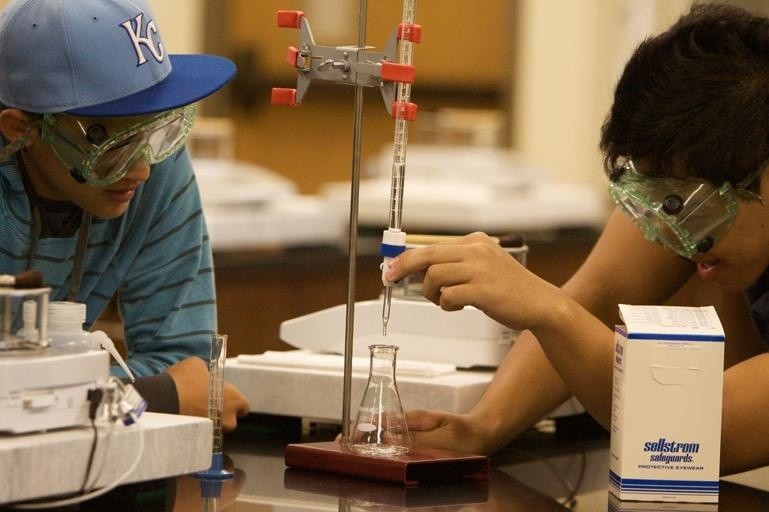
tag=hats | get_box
[0,0,240,120]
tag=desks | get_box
[64,415,769,511]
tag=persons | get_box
[336,0,769,477]
[0,0,250,434]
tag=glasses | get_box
[36,101,198,188]
[606,155,769,259]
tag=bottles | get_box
[194,330,231,479]
[349,343,412,458]
